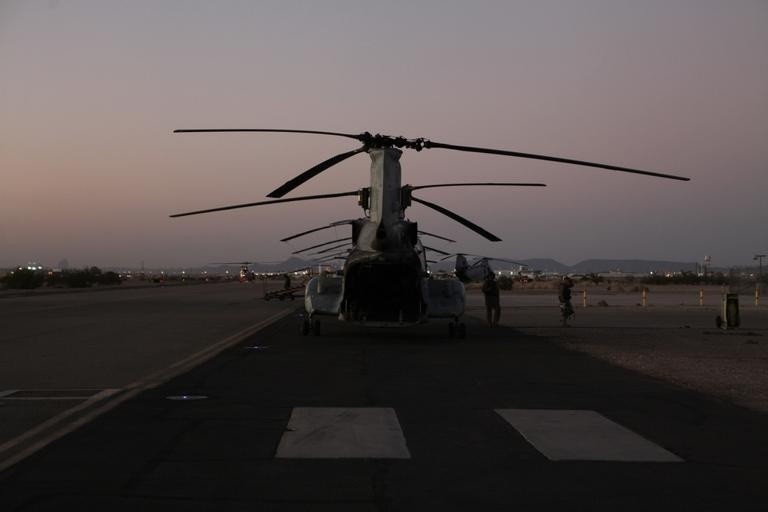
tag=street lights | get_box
[755,254,766,276]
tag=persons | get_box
[283,274,295,301]
[481,271,505,326]
[558,277,574,328]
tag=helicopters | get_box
[172,127,693,340]
[168,181,548,301]
